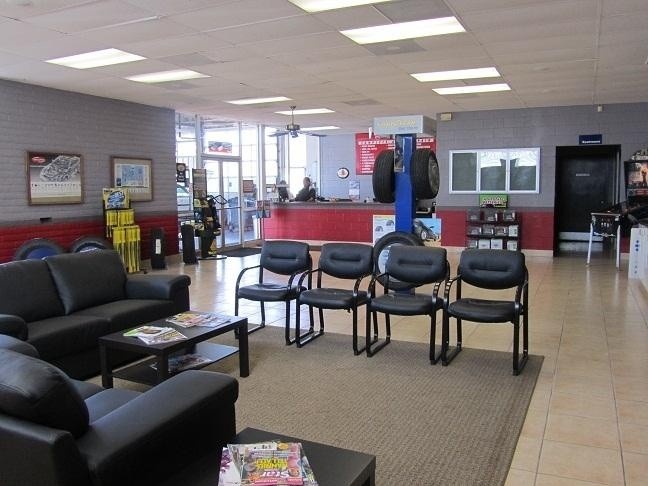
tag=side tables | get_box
[166,428,378,486]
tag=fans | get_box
[268,124,329,140]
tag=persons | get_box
[289,177,317,202]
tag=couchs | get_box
[1,335,242,486]
[1,248,193,380]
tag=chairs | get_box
[232,239,315,346]
[12,238,66,259]
[69,235,110,255]
[365,244,452,367]
[440,248,531,377]
[586,211,623,269]
[294,242,380,357]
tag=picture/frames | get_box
[22,149,87,207]
[108,155,155,204]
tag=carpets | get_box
[85,304,545,486]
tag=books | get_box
[149,352,212,374]
[123,326,176,339]
[165,313,230,328]
[217,438,319,486]
[137,327,188,345]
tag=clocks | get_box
[337,167,350,180]
